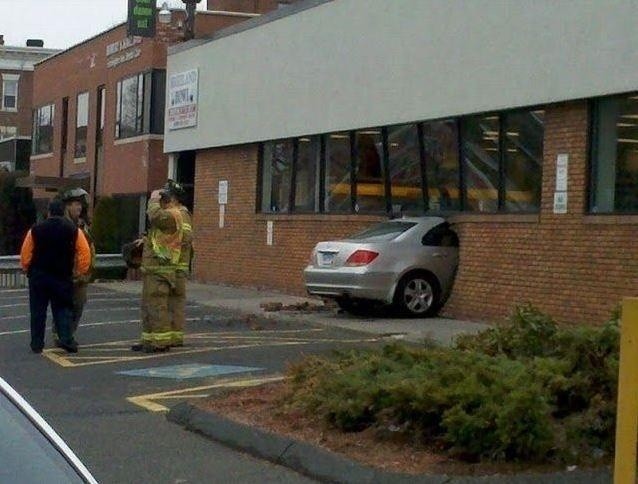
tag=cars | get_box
[304,215,459,317]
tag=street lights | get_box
[157,0,204,39]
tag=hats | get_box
[167,179,190,202]
[123,241,143,271]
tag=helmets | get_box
[60,186,89,203]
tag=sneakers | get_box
[31,346,44,354]
[131,341,184,354]
[53,339,80,354]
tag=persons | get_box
[161,179,194,348]
[51,187,96,347]
[131,188,184,354]
[19,196,91,353]
